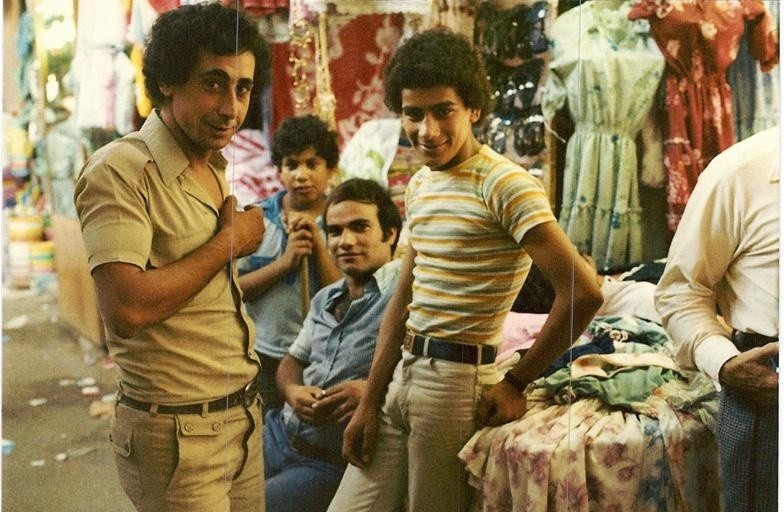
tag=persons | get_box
[232,112,344,428]
[264,178,407,512]
[323,25,606,510]
[73,0,268,512]
[651,121,778,511]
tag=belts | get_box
[283,433,346,469]
[402,333,497,365]
[732,328,774,352]
[119,379,261,415]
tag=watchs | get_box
[503,369,537,398]
